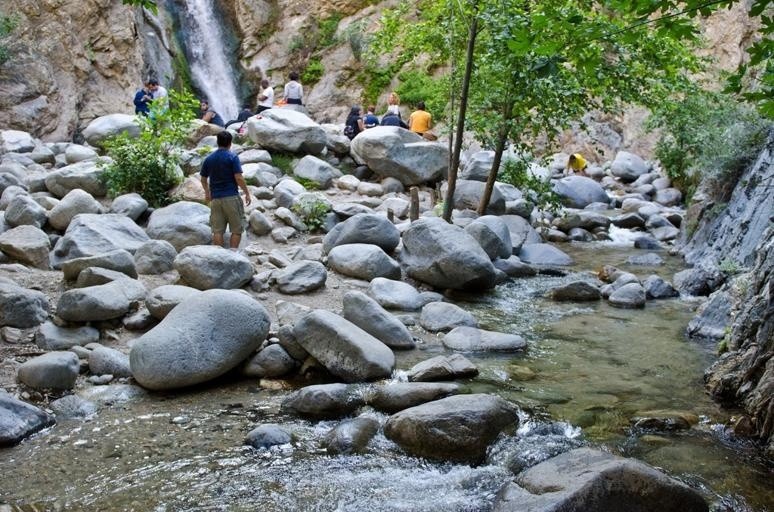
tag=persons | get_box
[133,78,176,137]
[343,92,433,141]
[200,99,224,128]
[283,72,304,105]
[238,103,254,122]
[565,153,589,177]
[255,79,274,114]
[199,131,252,252]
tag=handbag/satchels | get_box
[345,126,355,137]
[423,131,437,141]
